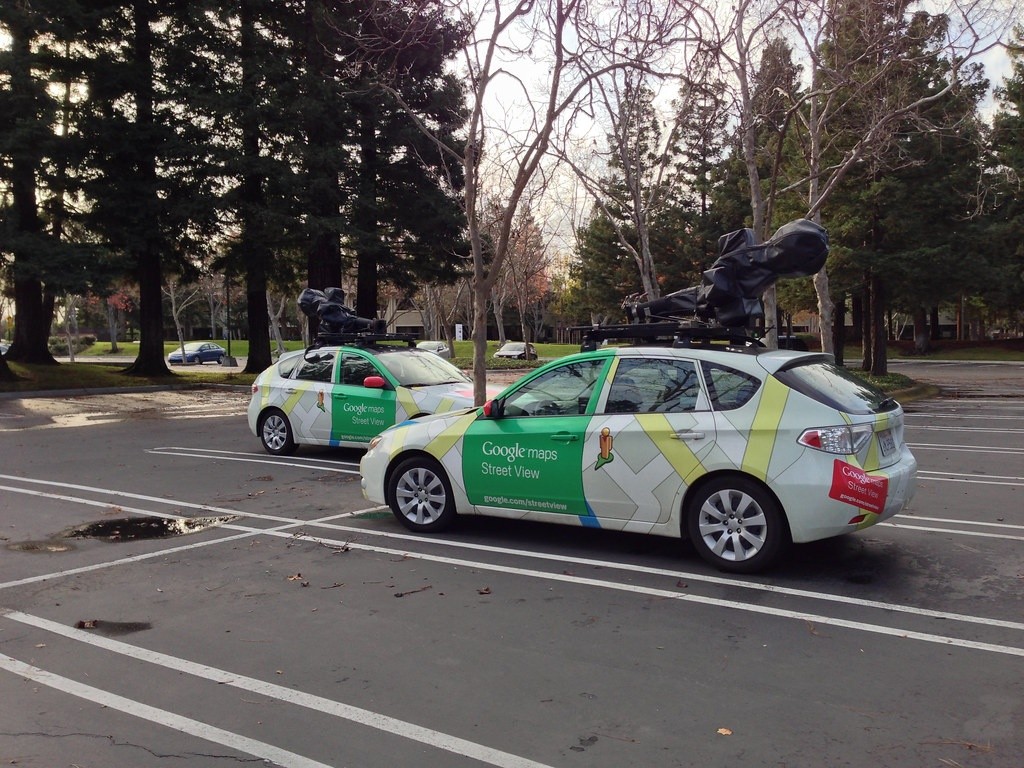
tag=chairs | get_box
[605,376,642,413]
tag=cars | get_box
[415,340,450,359]
[359,321,917,575]
[725,335,809,352]
[247,331,537,457]
[493,342,538,361]
[167,342,226,365]
